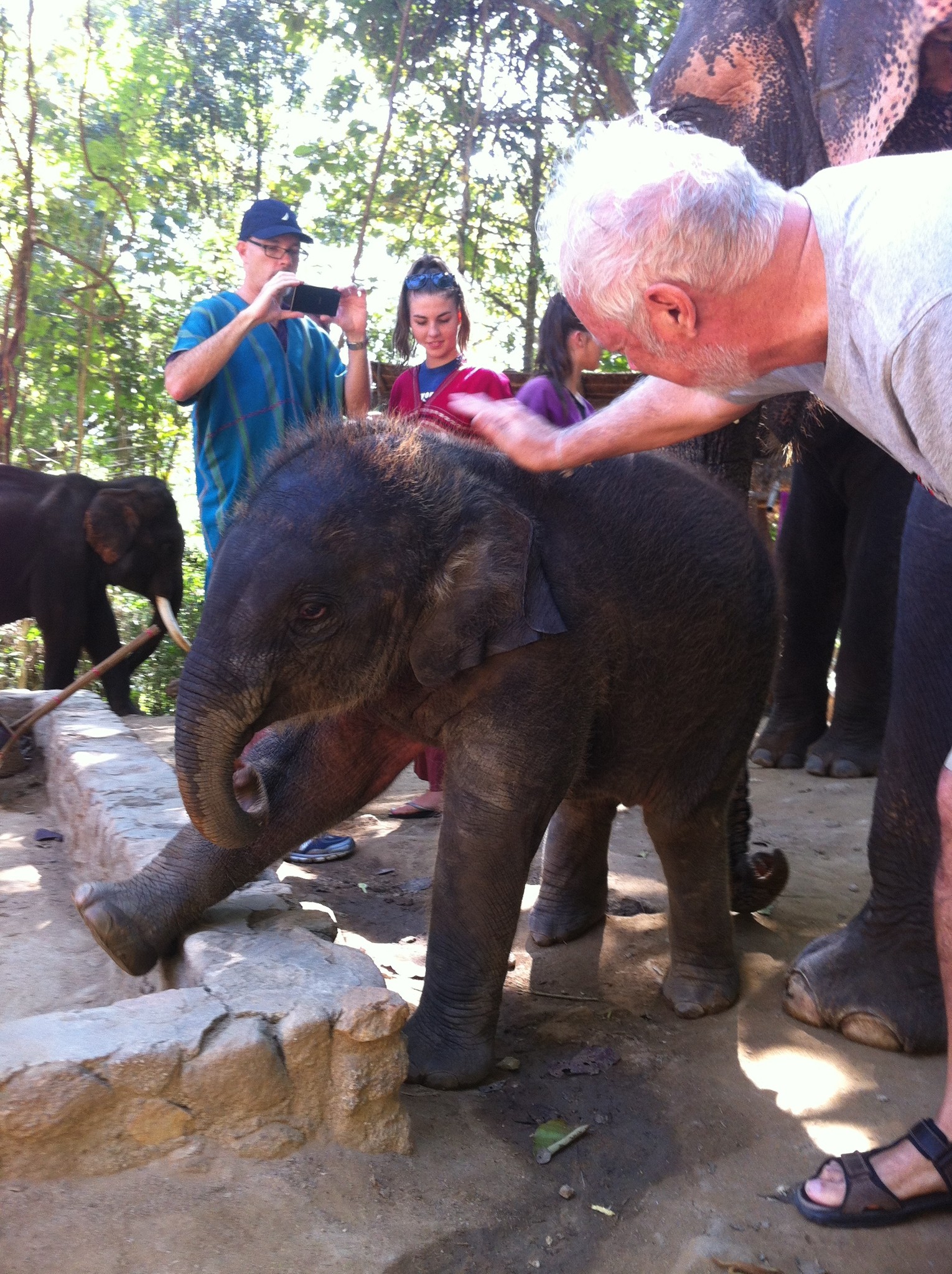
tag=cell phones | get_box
[290,284,342,317]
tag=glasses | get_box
[245,237,309,263]
[404,271,453,290]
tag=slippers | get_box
[389,801,443,819]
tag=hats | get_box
[239,199,314,244]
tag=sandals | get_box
[795,1115,952,1229]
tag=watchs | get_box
[345,335,369,353]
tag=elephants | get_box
[0,463,193,717]
[75,414,783,1091]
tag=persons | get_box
[451,110,952,1231]
[513,292,608,426]
[389,256,512,820]
[164,198,374,864]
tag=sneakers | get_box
[285,831,355,863]
